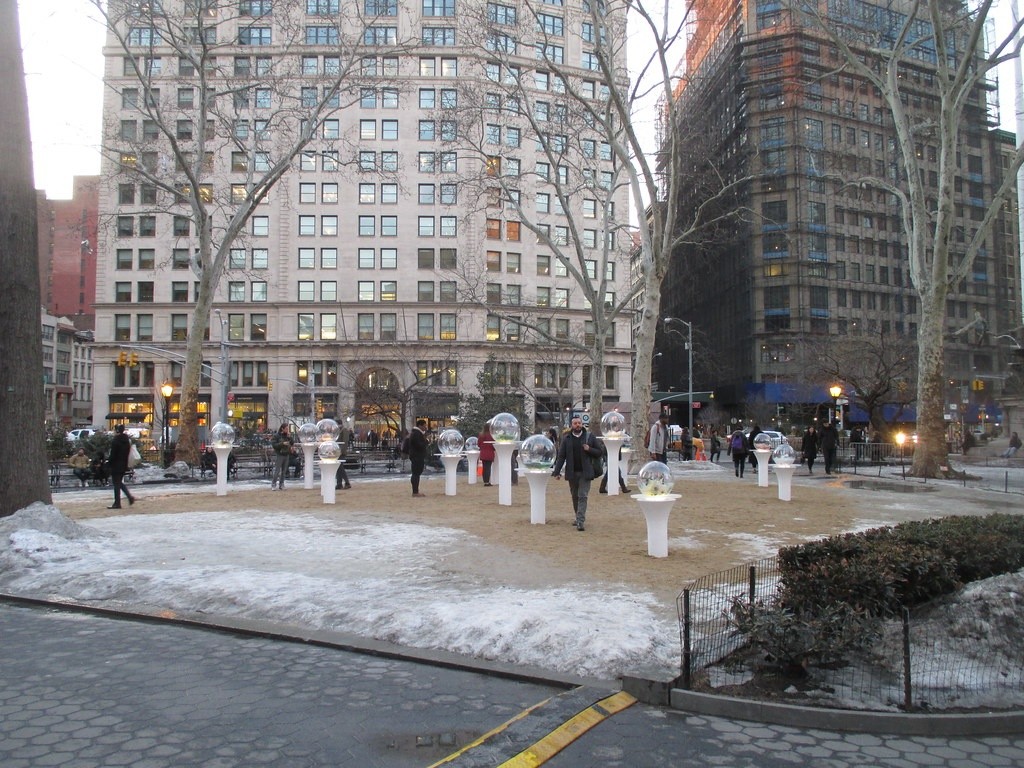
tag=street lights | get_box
[830,385,842,429]
[160,383,175,450]
[665,317,693,437]
[215,309,229,424]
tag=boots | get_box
[620,481,631,493]
[600,479,608,493]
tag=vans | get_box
[69,429,93,440]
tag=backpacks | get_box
[732,434,743,451]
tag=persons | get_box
[511,449,519,485]
[478,423,495,486]
[962,431,975,455]
[599,446,631,493]
[69,448,109,487]
[682,427,700,461]
[711,431,721,463]
[801,419,839,474]
[335,420,351,489]
[409,420,428,495]
[546,417,602,531]
[271,424,294,491]
[107,424,136,509]
[1000,431,1022,458]
[204,447,236,480]
[349,429,398,451]
[285,449,300,478]
[727,425,763,478]
[649,414,670,465]
[850,426,881,461]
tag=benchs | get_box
[48,464,134,489]
[202,451,402,482]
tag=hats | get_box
[114,423,125,433]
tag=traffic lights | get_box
[317,400,322,410]
[130,351,139,367]
[118,352,127,366]
[979,380,984,390]
[268,382,272,392]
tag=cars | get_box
[668,434,705,455]
[745,431,791,449]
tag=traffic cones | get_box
[695,448,702,460]
[701,446,707,461]
[199,441,204,450]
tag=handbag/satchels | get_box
[644,424,659,448]
[401,438,413,454]
[127,438,140,467]
[800,452,805,464]
[585,432,603,478]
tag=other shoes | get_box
[280,485,287,491]
[735,468,738,477]
[84,482,90,487]
[753,469,757,472]
[343,484,351,489]
[107,503,122,509]
[411,492,425,498]
[576,523,585,531]
[79,482,83,487]
[573,520,577,526]
[335,484,341,489]
[271,486,276,490]
[826,470,831,475]
[129,495,135,505]
[739,471,744,478]
